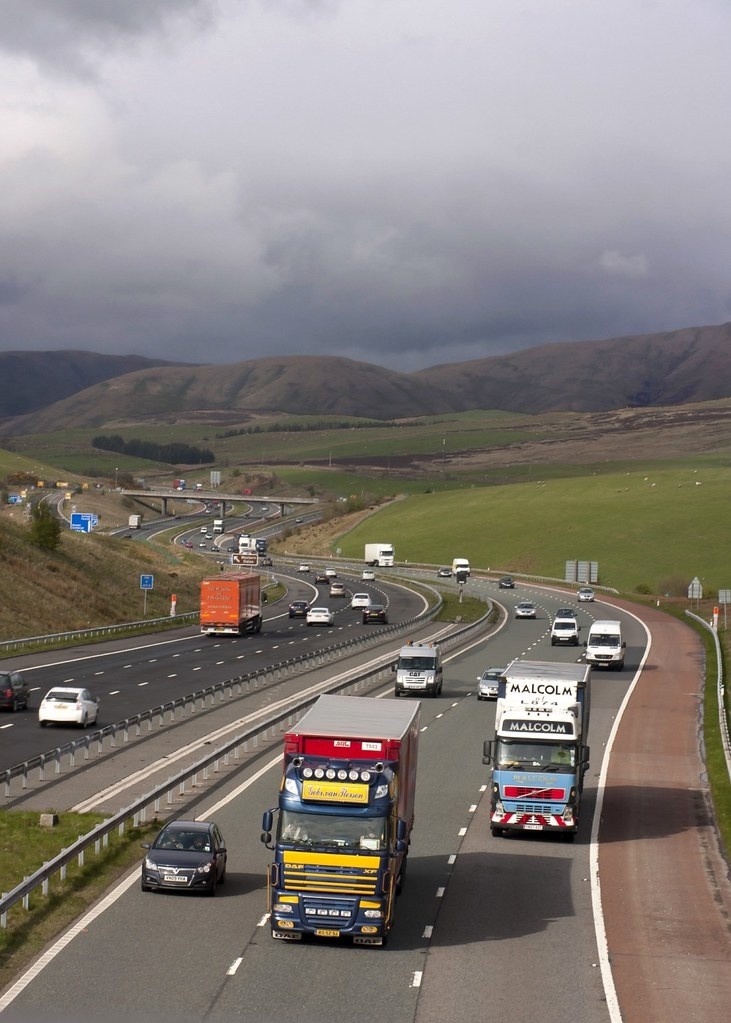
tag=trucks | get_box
[391,640,444,697]
[199,572,263,638]
[583,620,626,670]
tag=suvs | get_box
[549,618,581,646]
[288,600,313,618]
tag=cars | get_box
[361,605,388,625]
[123,477,471,611]
[498,576,515,588]
[476,667,505,701]
[38,686,100,728]
[514,602,537,620]
[553,608,578,618]
[0,670,31,712]
[306,607,335,626]
[577,587,596,602]
[140,819,227,895]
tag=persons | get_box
[551,745,570,764]
[161,833,184,849]
[361,822,384,840]
[189,836,205,850]
[284,817,308,838]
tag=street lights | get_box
[115,467,119,487]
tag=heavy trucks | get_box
[261,691,422,948]
[481,659,593,839]
